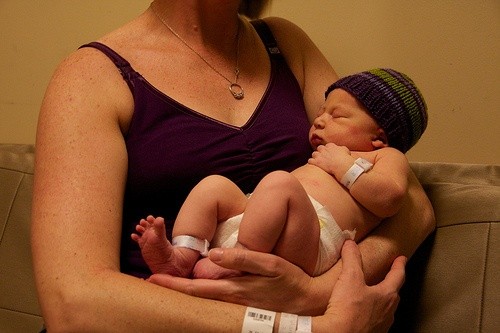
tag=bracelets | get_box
[241,306,312,333]
[340,158,373,189]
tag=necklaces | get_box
[143,2,245,99]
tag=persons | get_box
[131,67,428,280]
[29,0,435,333]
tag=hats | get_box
[324,68,429,155]
